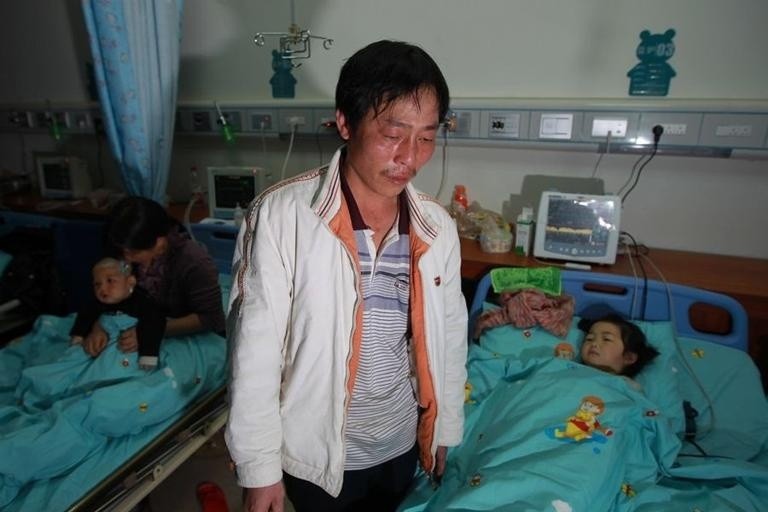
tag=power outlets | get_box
[652,123,688,136]
[717,124,752,138]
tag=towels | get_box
[491,266,561,299]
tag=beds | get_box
[0,221,242,510]
[404,268,766,511]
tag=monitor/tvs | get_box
[35,156,97,200]
[207,166,265,219]
[533,190,622,265]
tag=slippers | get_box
[196,480,229,512]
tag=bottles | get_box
[451,185,468,238]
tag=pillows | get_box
[480,301,685,433]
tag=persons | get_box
[83,194,227,357]
[218,40,470,511]
[68,251,168,371]
[580,310,661,394]
